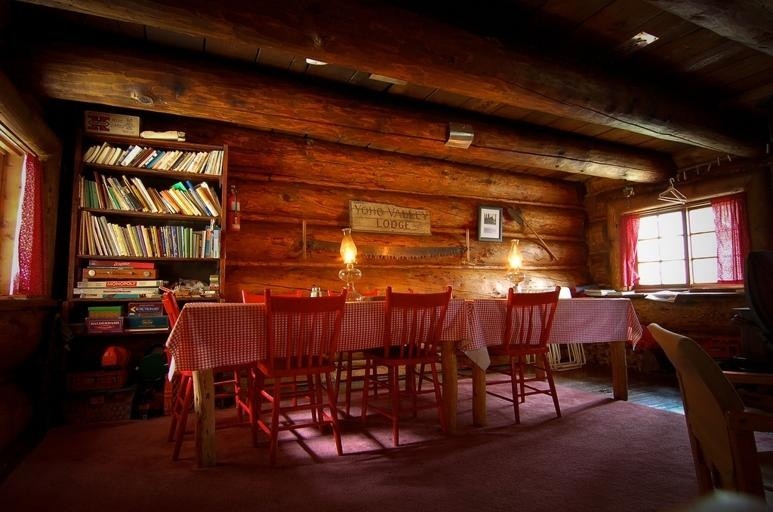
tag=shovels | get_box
[504,206,559,263]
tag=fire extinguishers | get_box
[227,184,242,235]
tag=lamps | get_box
[506,239,526,293]
[339,228,364,302]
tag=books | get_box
[73,140,225,300]
[584,289,658,296]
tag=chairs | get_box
[360,286,452,446]
[161,291,260,463]
[327,289,412,418]
[253,288,348,465]
[486,286,568,424]
[241,288,318,424]
[646,322,773,512]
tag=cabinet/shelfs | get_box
[61,127,229,337]
[477,205,503,242]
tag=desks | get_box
[181,302,459,467]
[468,297,631,426]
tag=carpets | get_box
[0,372,772,512]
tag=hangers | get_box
[658,176,687,206]
